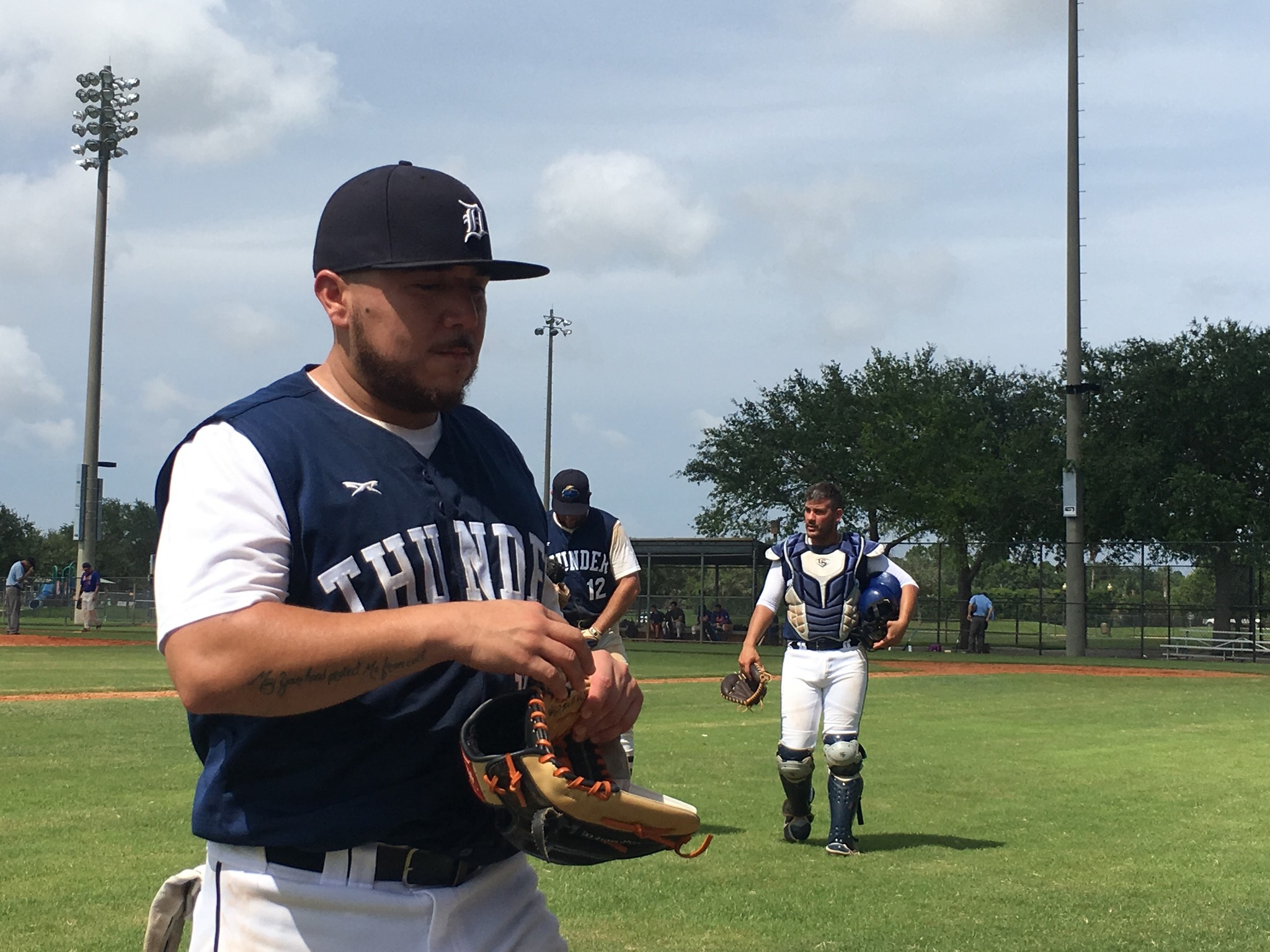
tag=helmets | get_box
[859,571,912,651]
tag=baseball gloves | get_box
[76,598,82,609]
[719,663,767,706]
[458,677,700,867]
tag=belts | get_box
[572,619,594,630]
[788,638,859,652]
[265,837,492,889]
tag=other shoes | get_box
[825,826,855,856]
[784,786,817,844]
[962,649,971,654]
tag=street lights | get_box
[70,63,142,626]
[534,308,573,505]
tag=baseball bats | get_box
[902,628,918,650]
[955,633,960,652]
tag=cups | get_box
[908,645,912,652]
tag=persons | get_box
[6,558,35,635]
[738,483,919,855]
[691,604,732,641]
[546,469,641,775]
[650,601,686,638]
[965,592,993,653]
[156,161,644,952]
[78,562,102,631]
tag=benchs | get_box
[619,625,747,641]
[1160,632,1270,661]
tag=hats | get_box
[311,161,553,287]
[550,468,596,515]
